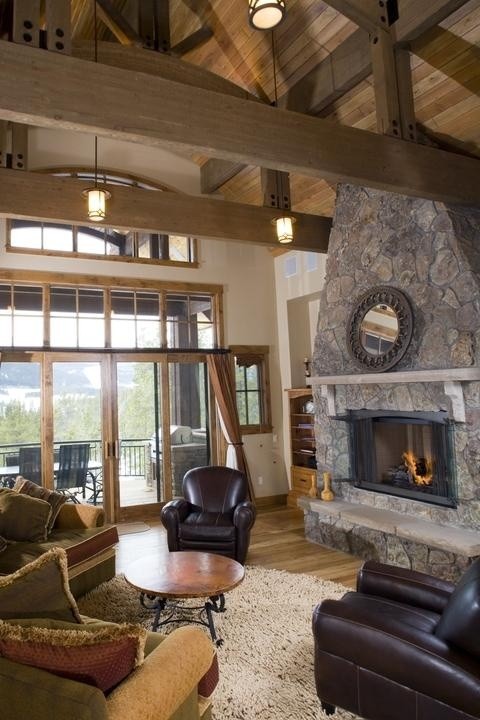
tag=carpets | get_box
[76,563,365,720]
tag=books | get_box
[300,449,315,453]
[299,423,314,428]
[301,437,315,441]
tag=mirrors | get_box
[346,284,415,373]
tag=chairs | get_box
[0,503,119,602]
[6,456,19,467]
[53,444,90,503]
[0,613,220,720]
[53,452,59,463]
[11,447,42,486]
[159,466,256,567]
[312,553,480,720]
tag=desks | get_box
[0,461,103,505]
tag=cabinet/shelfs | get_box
[284,388,317,509]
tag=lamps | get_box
[248,0,286,31]
[80,0,112,221]
[271,28,298,244]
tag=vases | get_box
[308,472,335,502]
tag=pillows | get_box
[0,546,84,624]
[10,476,70,535]
[0,486,52,543]
[0,618,147,693]
